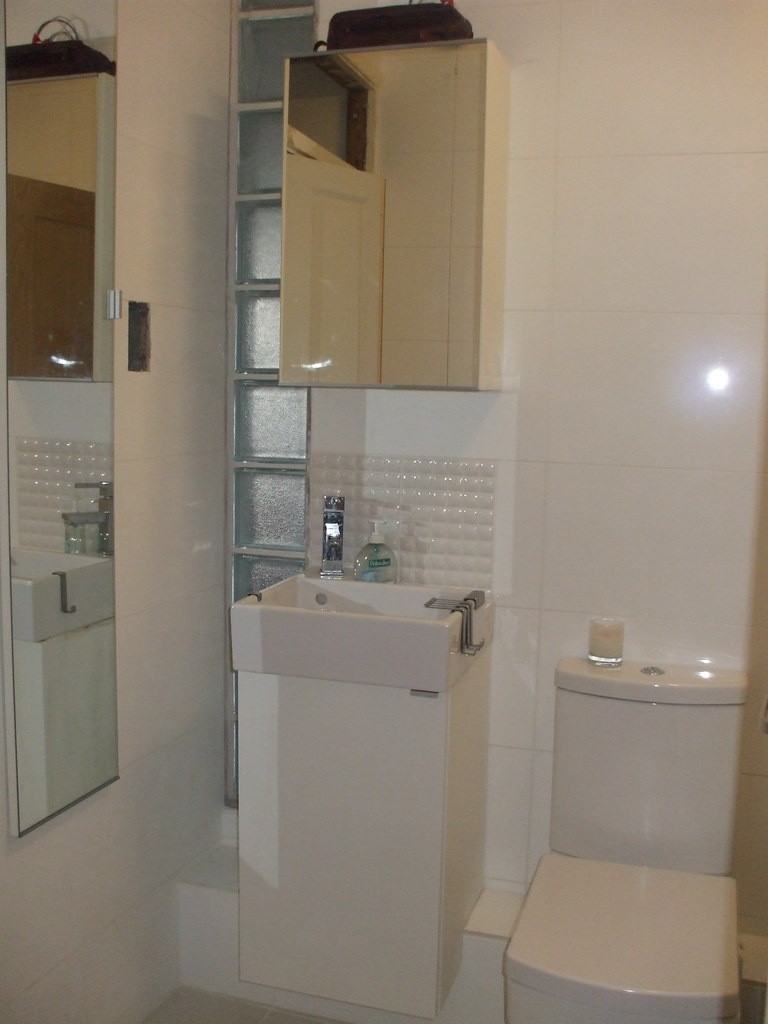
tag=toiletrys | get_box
[353,521,398,584]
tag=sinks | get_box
[11,545,114,643]
[230,574,494,692]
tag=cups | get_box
[588,617,625,668]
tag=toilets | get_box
[504,656,750,1024]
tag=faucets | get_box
[62,481,113,556]
[320,496,348,578]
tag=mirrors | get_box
[4,0,121,837]
[278,37,509,390]
